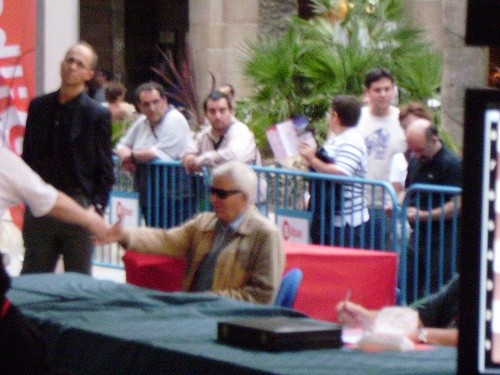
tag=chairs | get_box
[276,270,302,307]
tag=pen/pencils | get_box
[338,290,351,322]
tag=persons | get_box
[182,90,268,216]
[92,162,285,304]
[0,140,122,317]
[85,70,137,187]
[327,68,408,251]
[19,41,116,276]
[384,100,462,305]
[335,276,458,348]
[299,95,370,249]
[116,82,203,229]
[219,83,234,103]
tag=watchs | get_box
[418,328,427,344]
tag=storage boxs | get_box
[218,316,342,351]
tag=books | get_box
[265,117,317,163]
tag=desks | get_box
[6,260,457,375]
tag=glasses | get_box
[209,185,241,199]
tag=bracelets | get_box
[130,149,135,161]
